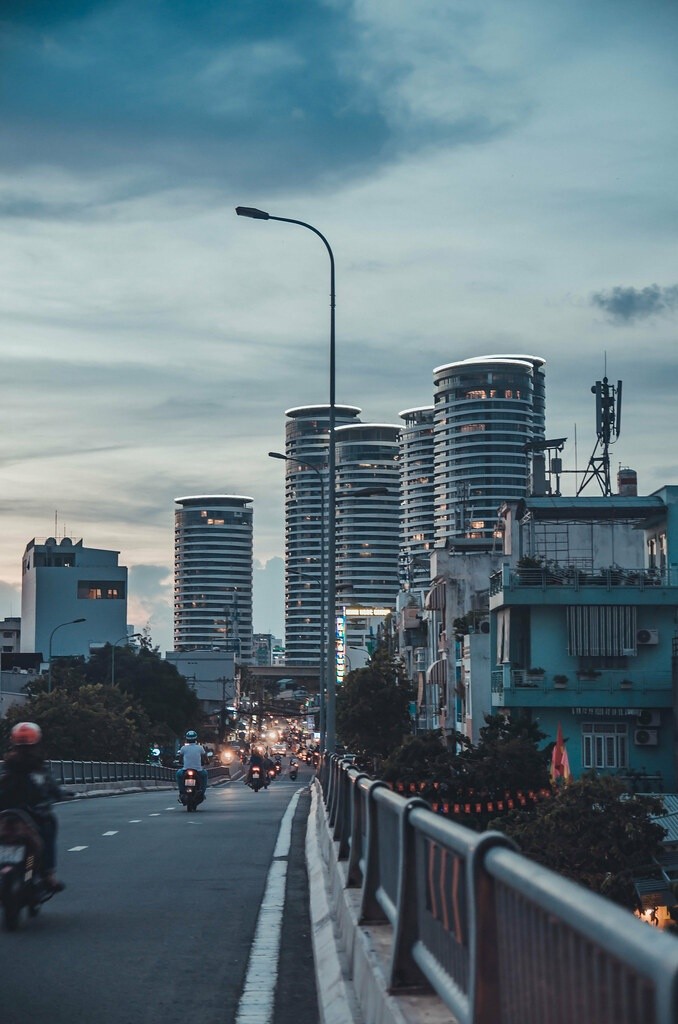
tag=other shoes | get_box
[42,879,66,892]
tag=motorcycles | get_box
[265,760,298,790]
[307,756,318,769]
[1,790,77,931]
[248,766,267,793]
[174,750,213,813]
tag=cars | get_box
[211,711,313,765]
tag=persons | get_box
[175,730,208,804]
[275,753,281,762]
[245,749,275,781]
[0,722,65,893]
[289,753,297,765]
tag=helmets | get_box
[13,723,41,745]
[186,731,197,740]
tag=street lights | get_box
[48,617,86,692]
[111,633,142,687]
[268,451,326,757]
[236,206,338,756]
